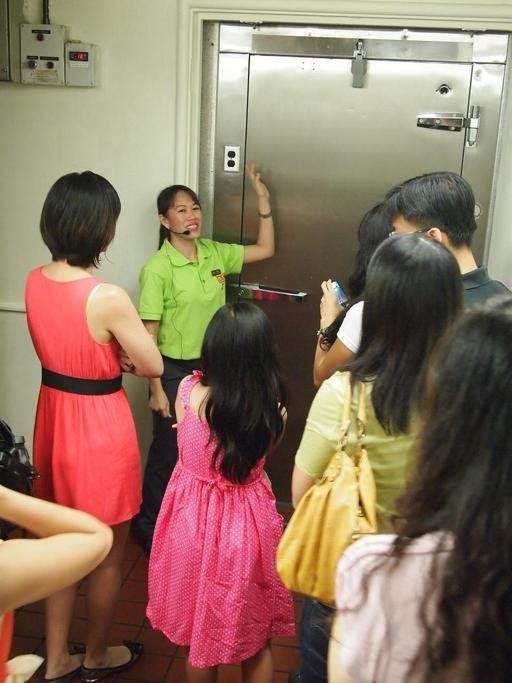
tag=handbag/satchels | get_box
[276,372,378,606]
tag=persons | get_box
[131,162,276,555]
[25,170,165,681]
[275,170,511,683]
[1,483,113,683]
[147,302,296,682]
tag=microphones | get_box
[163,226,191,236]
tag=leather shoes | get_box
[44,645,86,683]
[79,641,144,683]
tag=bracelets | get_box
[261,212,275,220]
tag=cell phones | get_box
[325,279,350,308]
